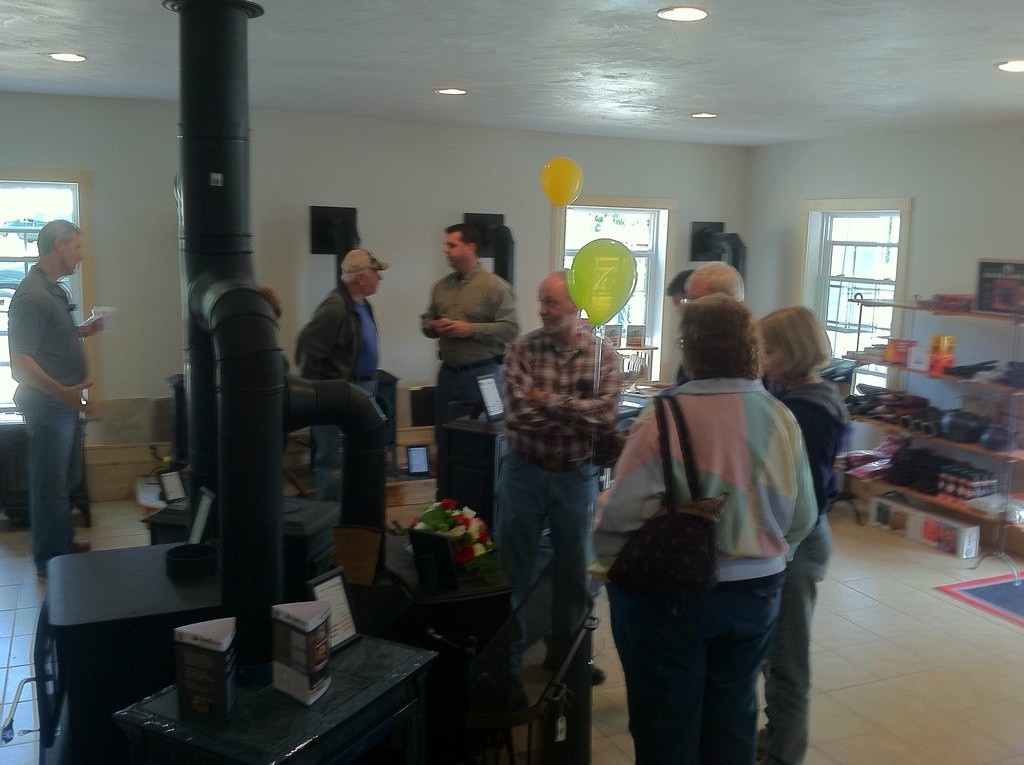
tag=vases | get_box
[403,525,472,586]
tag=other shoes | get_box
[36,570,49,582]
[591,662,607,685]
[70,536,92,553]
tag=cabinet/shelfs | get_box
[110,632,438,765]
[841,291,1024,557]
[373,534,515,765]
[42,541,224,765]
[140,489,341,612]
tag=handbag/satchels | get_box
[604,394,732,619]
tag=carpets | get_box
[931,568,1024,627]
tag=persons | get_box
[754,307,853,765]
[594,293,819,764]
[260,287,289,375]
[422,223,519,502]
[294,251,387,502]
[7,218,108,582]
[667,268,691,302]
[505,266,623,685]
[676,260,746,382]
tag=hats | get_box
[342,249,388,273]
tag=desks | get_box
[0,400,104,529]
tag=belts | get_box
[443,357,499,373]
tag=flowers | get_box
[408,497,499,580]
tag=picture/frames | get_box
[156,468,186,505]
[627,325,646,347]
[604,324,621,347]
[406,443,431,475]
[304,566,363,658]
[473,373,506,423]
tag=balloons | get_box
[541,157,583,206]
[568,236,638,330]
